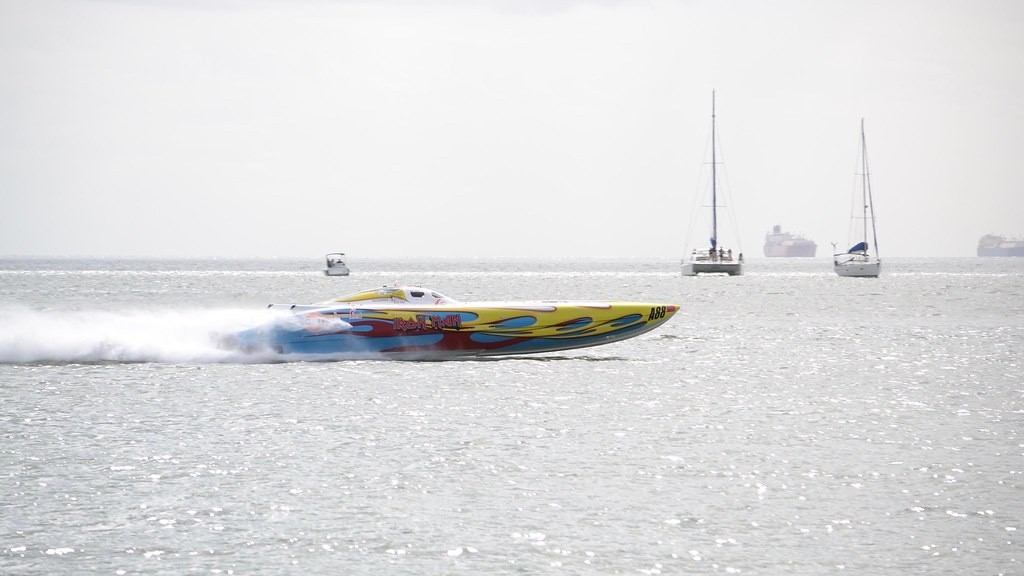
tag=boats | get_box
[977,234,1024,257]
[324,252,350,276]
[755,224,818,271]
[219,286,682,350]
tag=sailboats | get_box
[679,88,744,275]
[831,117,882,278]
